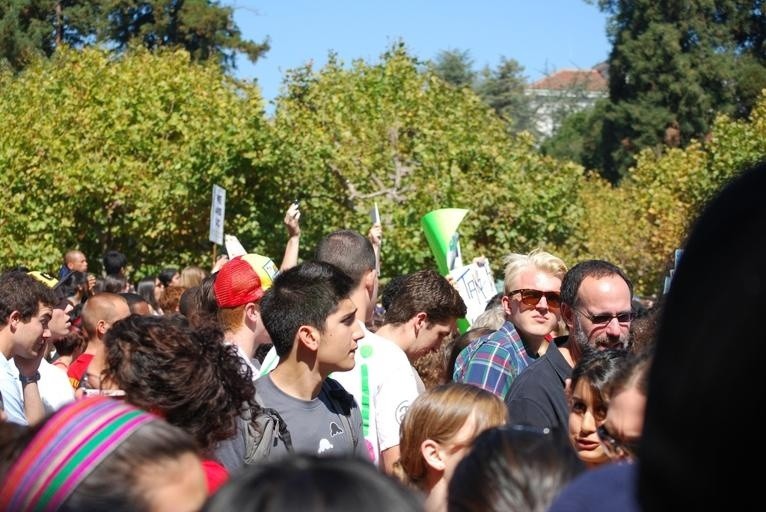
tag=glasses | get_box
[596,424,640,464]
[508,289,561,307]
[575,308,633,324]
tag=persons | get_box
[631,153,765,512]
[1,204,677,512]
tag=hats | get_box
[26,270,74,291]
[213,253,280,309]
